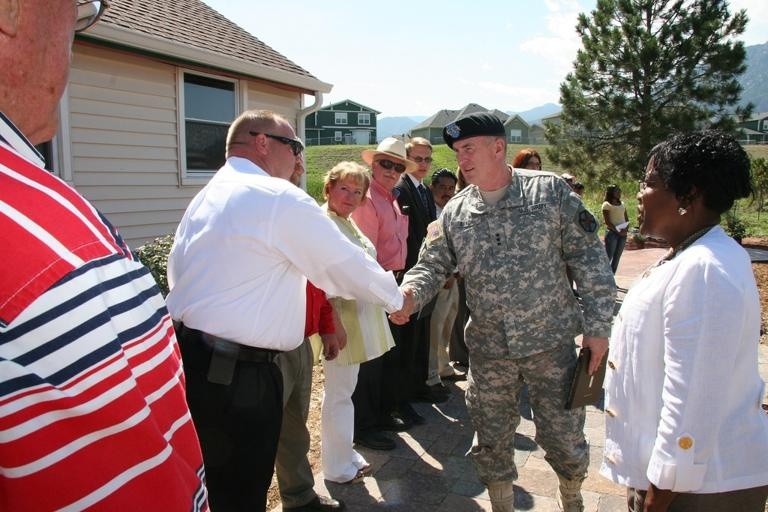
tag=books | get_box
[563,347,609,410]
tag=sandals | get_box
[343,471,364,484]
[358,464,372,473]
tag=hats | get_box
[574,181,584,189]
[443,113,506,152]
[362,137,418,173]
[561,173,575,179]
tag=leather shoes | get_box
[354,427,395,451]
[409,384,447,403]
[399,404,426,424]
[282,496,344,511]
[377,412,413,430]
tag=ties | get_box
[417,184,430,223]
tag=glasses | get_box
[409,156,433,163]
[377,159,406,172]
[638,179,666,194]
[75,1,108,32]
[250,131,304,156]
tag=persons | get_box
[449,164,471,367]
[387,113,616,512]
[601,184,629,275]
[512,148,542,171]
[0,0,213,512]
[166,110,414,512]
[348,137,413,450]
[561,173,575,190]
[574,183,584,195]
[274,137,343,512]
[425,168,466,395]
[599,130,768,512]
[308,160,397,483]
[395,136,449,425]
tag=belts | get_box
[172,321,276,363]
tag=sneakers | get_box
[441,371,466,380]
[428,383,451,393]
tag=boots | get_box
[558,474,584,512]
[488,482,515,512]
